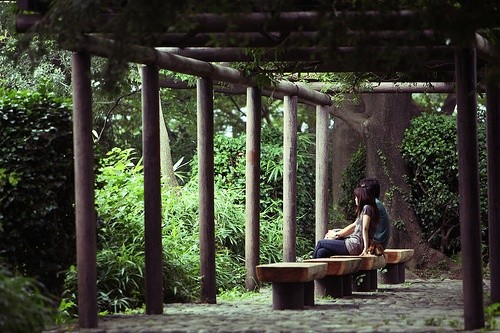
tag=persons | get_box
[311,178,391,258]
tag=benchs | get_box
[255,246,417,312]
